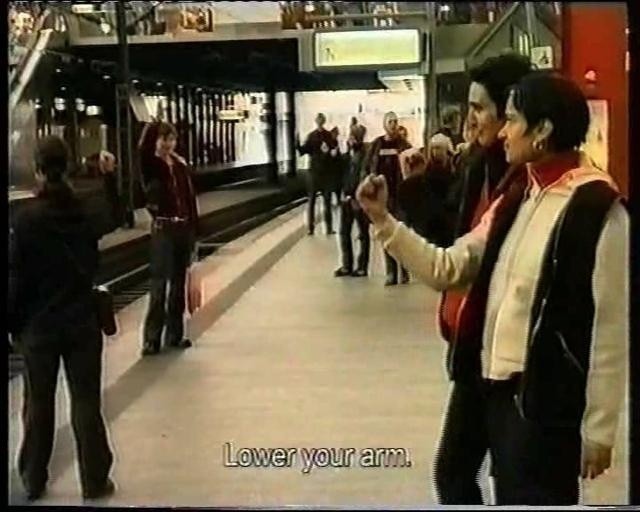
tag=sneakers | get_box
[307,230,410,286]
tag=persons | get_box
[355,70,632,504]
[135,101,202,356]
[431,50,538,503]
[2,134,132,504]
[293,104,476,288]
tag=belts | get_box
[152,217,187,226]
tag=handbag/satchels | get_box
[186,262,203,314]
[93,285,116,336]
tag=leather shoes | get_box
[164,337,191,349]
[26,484,46,502]
[82,477,115,499]
[141,343,161,355]
